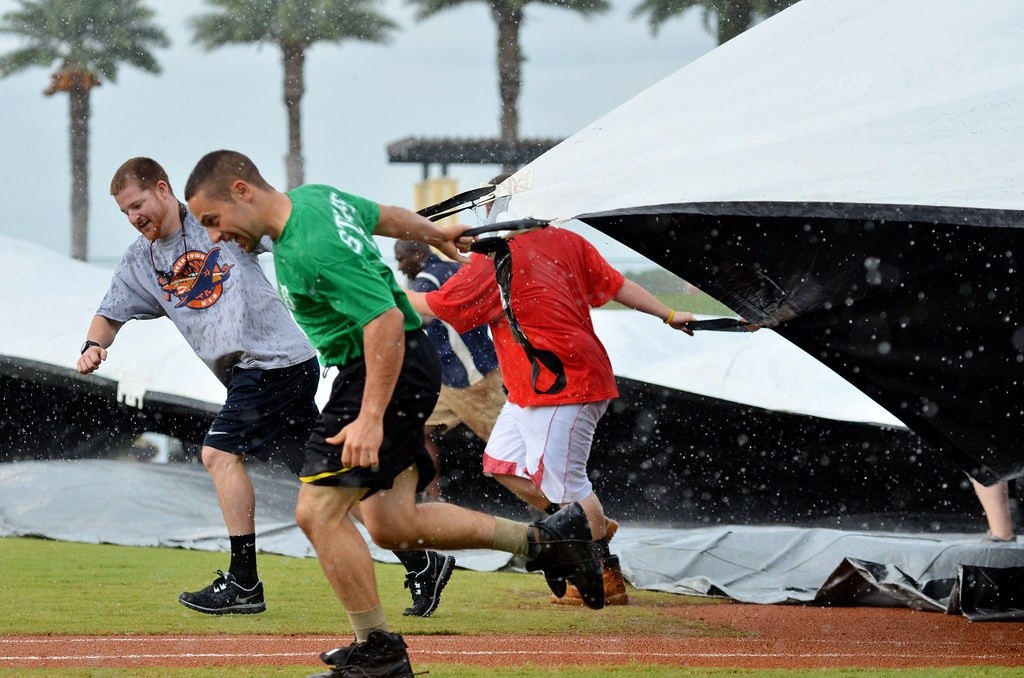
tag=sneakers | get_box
[603,514,619,543]
[308,628,429,678]
[179,569,266,615]
[524,500,606,610]
[402,549,456,618]
[549,555,629,605]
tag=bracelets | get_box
[663,309,675,324]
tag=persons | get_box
[77,156,456,619]
[963,467,1017,544]
[394,239,509,493]
[401,223,698,605]
[184,148,606,678]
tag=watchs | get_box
[80,340,101,355]
